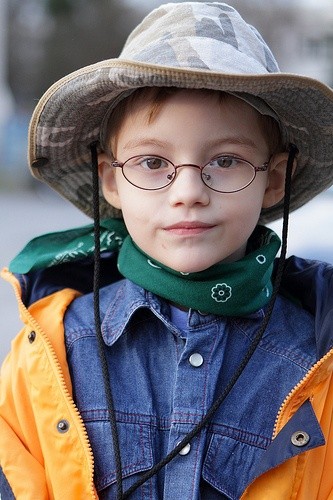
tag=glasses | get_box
[107,154,273,194]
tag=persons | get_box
[0,3,333,500]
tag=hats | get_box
[27,2,333,223]
